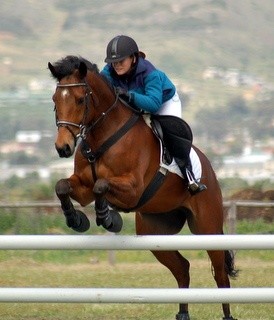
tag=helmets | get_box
[104,36,138,63]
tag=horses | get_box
[46,54,241,320]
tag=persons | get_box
[99,36,206,196]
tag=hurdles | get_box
[1,235,274,302]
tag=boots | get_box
[175,155,207,194]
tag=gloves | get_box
[116,86,128,98]
